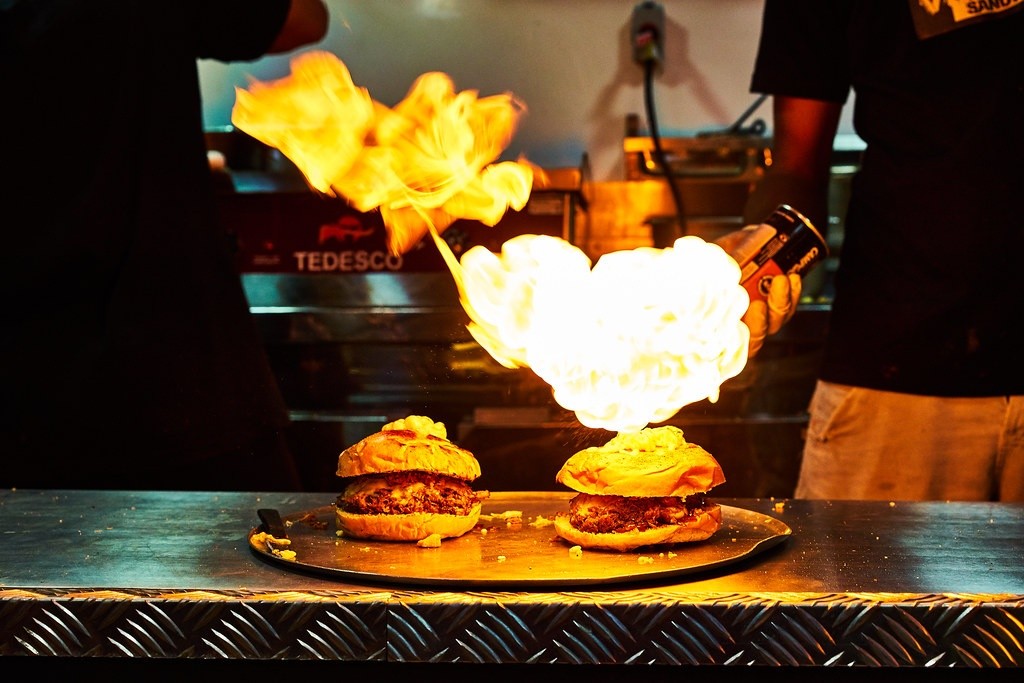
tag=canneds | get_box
[736,204,831,303]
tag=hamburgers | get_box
[332,415,488,548]
[555,425,727,550]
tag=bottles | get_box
[730,203,827,304]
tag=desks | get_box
[0,488,1024,683]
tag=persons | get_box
[717,0,1023,504]
[0,0,329,491]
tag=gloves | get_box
[713,224,803,359]
